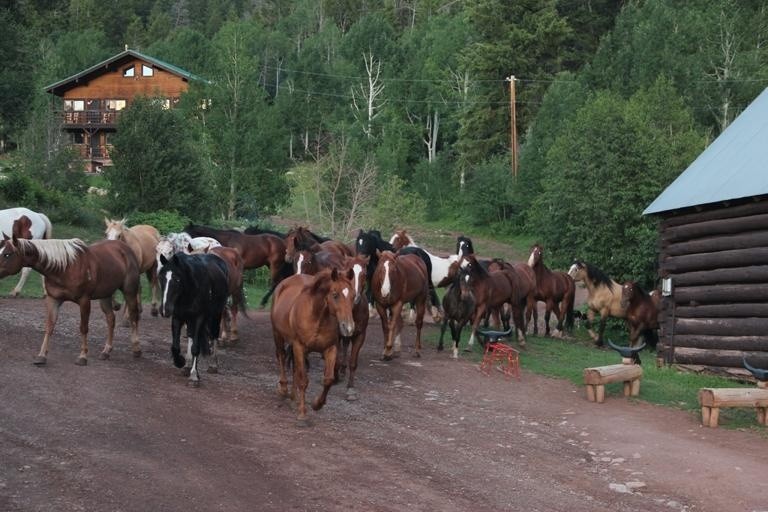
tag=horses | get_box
[154,220,663,428]
[103,215,162,328]
[0,230,142,367]
[0,207,52,299]
